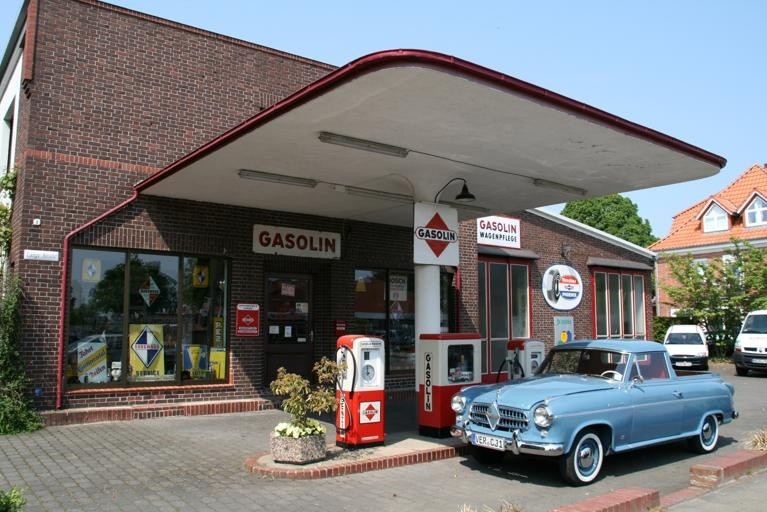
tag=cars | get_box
[64,333,123,383]
[381,322,414,384]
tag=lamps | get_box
[433,178,476,204]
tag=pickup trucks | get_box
[451,334,741,486]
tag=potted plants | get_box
[269,356,349,463]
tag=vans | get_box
[661,323,708,372]
[732,309,767,377]
